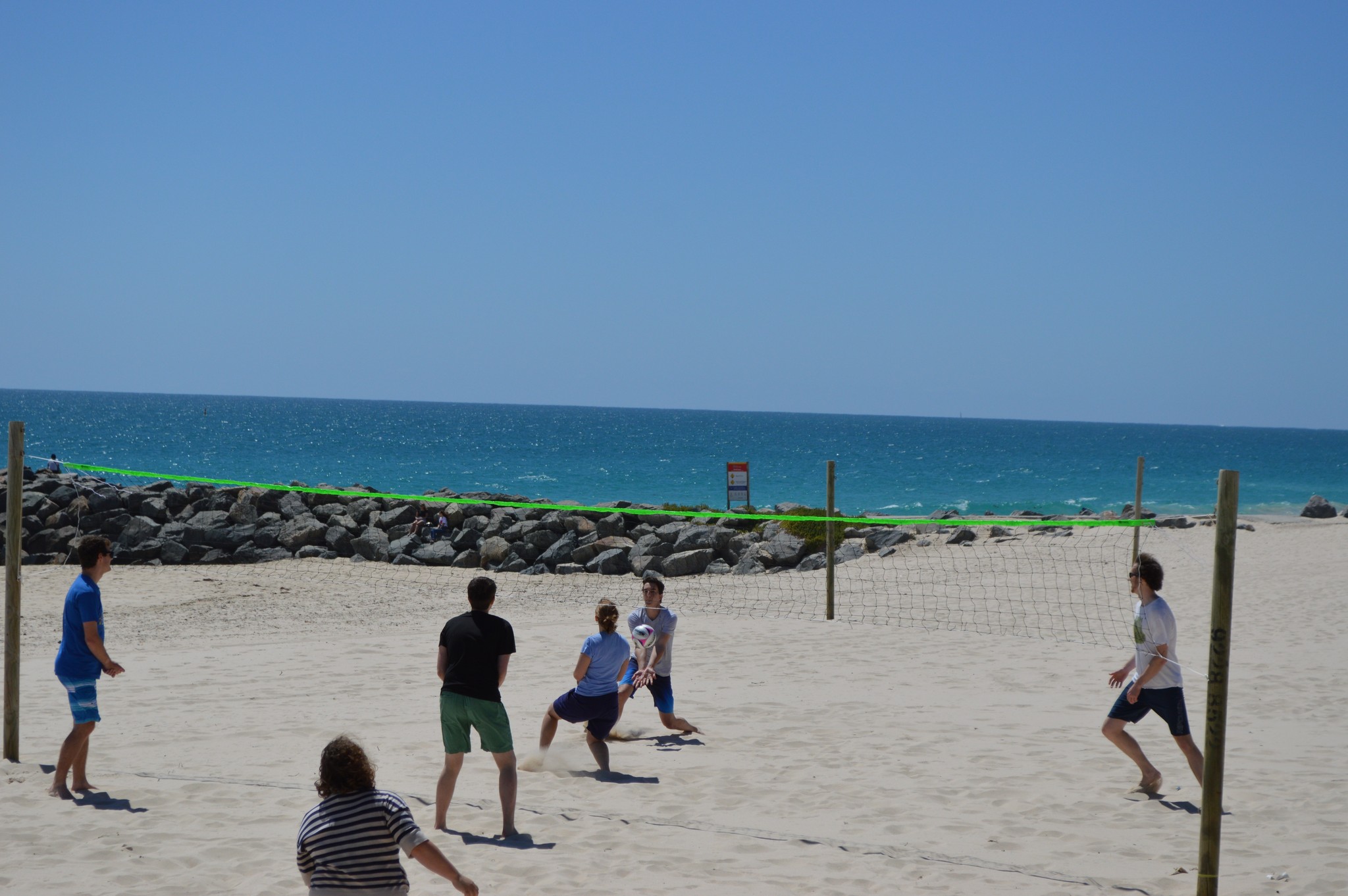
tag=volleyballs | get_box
[632,624,656,649]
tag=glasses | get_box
[102,550,114,558]
[1129,572,1138,578]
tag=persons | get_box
[617,576,705,736]
[430,510,449,543]
[48,454,60,474]
[296,734,479,896]
[517,598,631,773]
[1100,552,1205,794]
[47,535,125,800]
[407,502,429,536]
[433,576,519,838]
